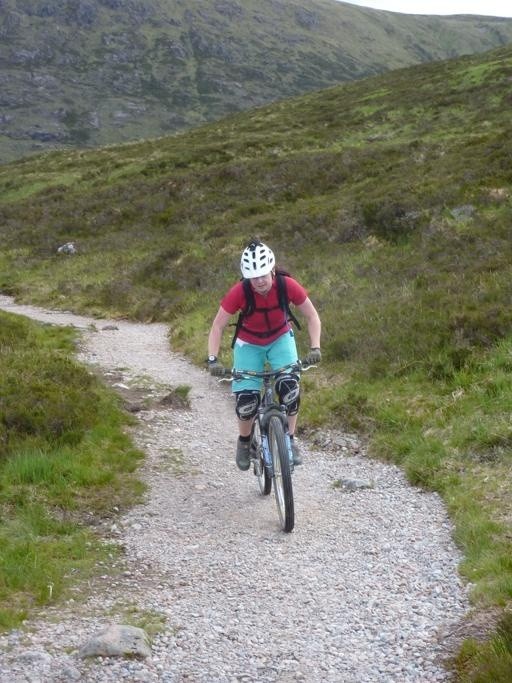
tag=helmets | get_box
[240,243,275,279]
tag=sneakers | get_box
[292,443,302,465]
[237,439,250,470]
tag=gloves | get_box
[210,362,225,376]
[306,348,321,364]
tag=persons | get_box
[208,241,321,471]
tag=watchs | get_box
[207,355,217,363]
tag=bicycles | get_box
[218,355,320,534]
